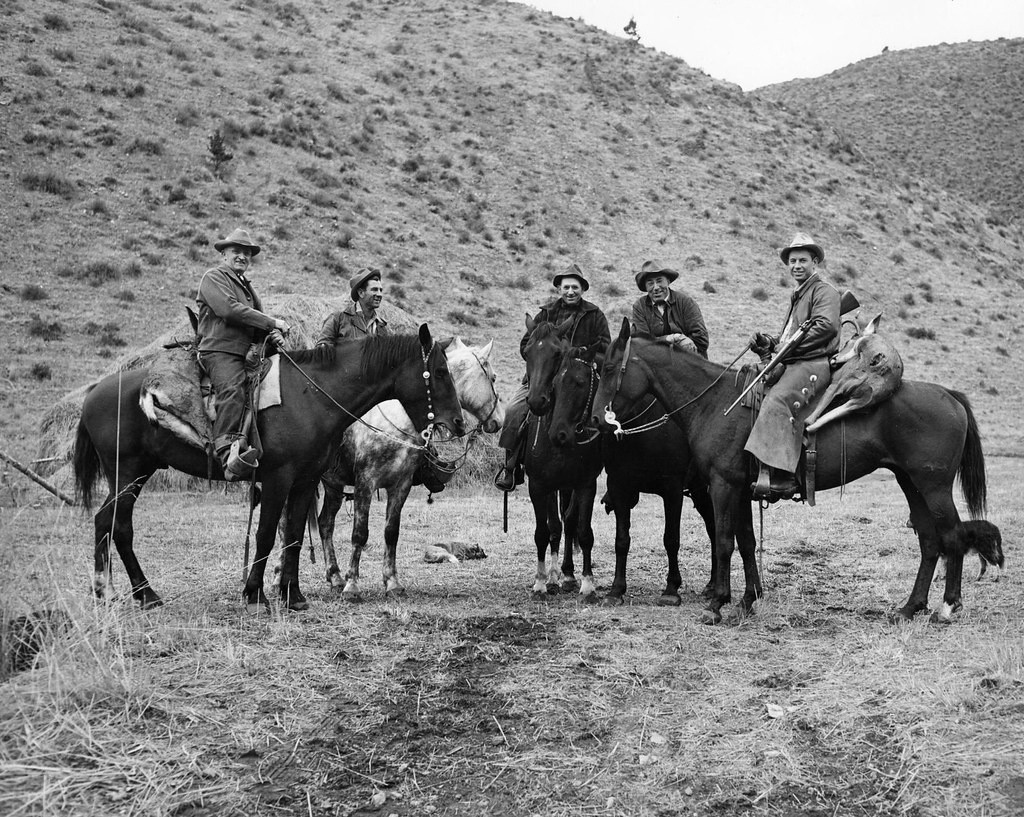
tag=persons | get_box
[633,258,709,360]
[314,268,388,347]
[195,227,291,482]
[744,232,841,494]
[496,263,612,489]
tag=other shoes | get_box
[494,469,513,489]
[224,448,259,481]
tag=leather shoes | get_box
[751,471,799,493]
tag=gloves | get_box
[748,331,768,353]
[270,331,285,347]
[677,333,697,353]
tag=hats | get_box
[214,227,261,256]
[635,259,679,291]
[349,268,381,301]
[552,263,589,291]
[780,233,825,266]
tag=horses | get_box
[517,305,990,624]
[71,325,507,613]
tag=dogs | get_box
[907,511,1006,582]
